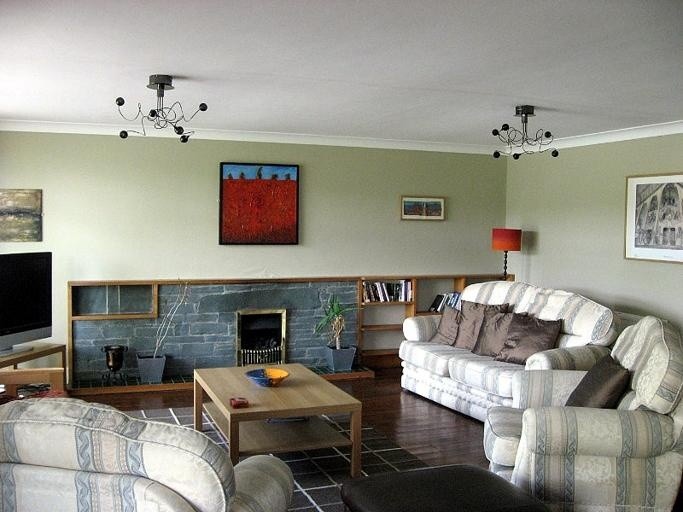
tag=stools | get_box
[337,461,554,512]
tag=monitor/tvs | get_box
[0,251,53,357]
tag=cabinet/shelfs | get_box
[0,339,66,407]
[352,272,517,367]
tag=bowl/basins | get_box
[245,367,289,388]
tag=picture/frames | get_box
[216,161,299,247]
[622,171,682,265]
[399,194,446,223]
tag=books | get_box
[360,279,412,303]
[426,292,459,314]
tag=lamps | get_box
[484,105,559,162]
[489,226,522,281]
[112,73,207,145]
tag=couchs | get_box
[395,280,625,427]
[477,312,682,511]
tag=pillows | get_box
[492,312,562,369]
[426,302,462,345]
[469,304,529,359]
[561,353,631,410]
[450,296,509,352]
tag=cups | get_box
[102,345,128,371]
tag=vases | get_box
[134,349,166,384]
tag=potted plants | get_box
[308,290,367,372]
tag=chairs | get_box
[0,395,298,512]
